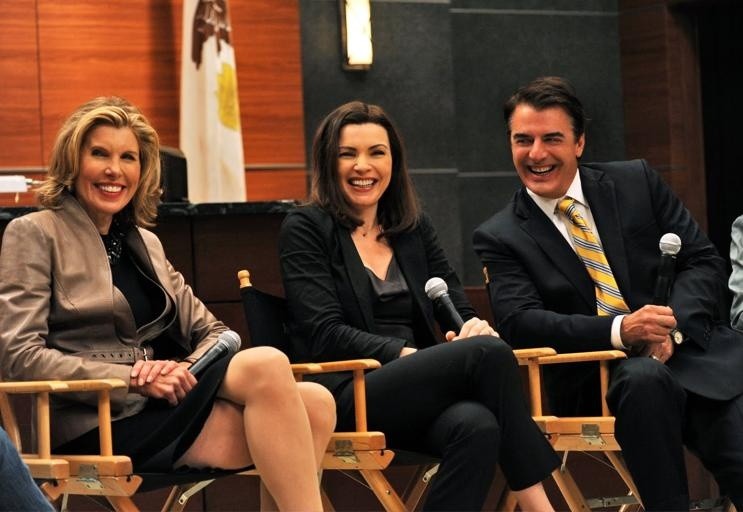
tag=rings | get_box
[649,353,659,360]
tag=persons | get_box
[0,93,339,512]
[276,98,565,512]
[472,73,743,511]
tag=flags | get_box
[176,1,249,209]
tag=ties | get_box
[556,197,632,315]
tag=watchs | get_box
[668,329,683,345]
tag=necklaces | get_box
[92,217,127,266]
[354,218,375,241]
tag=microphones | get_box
[187,330,242,380]
[424,277,464,336]
[652,233,682,306]
